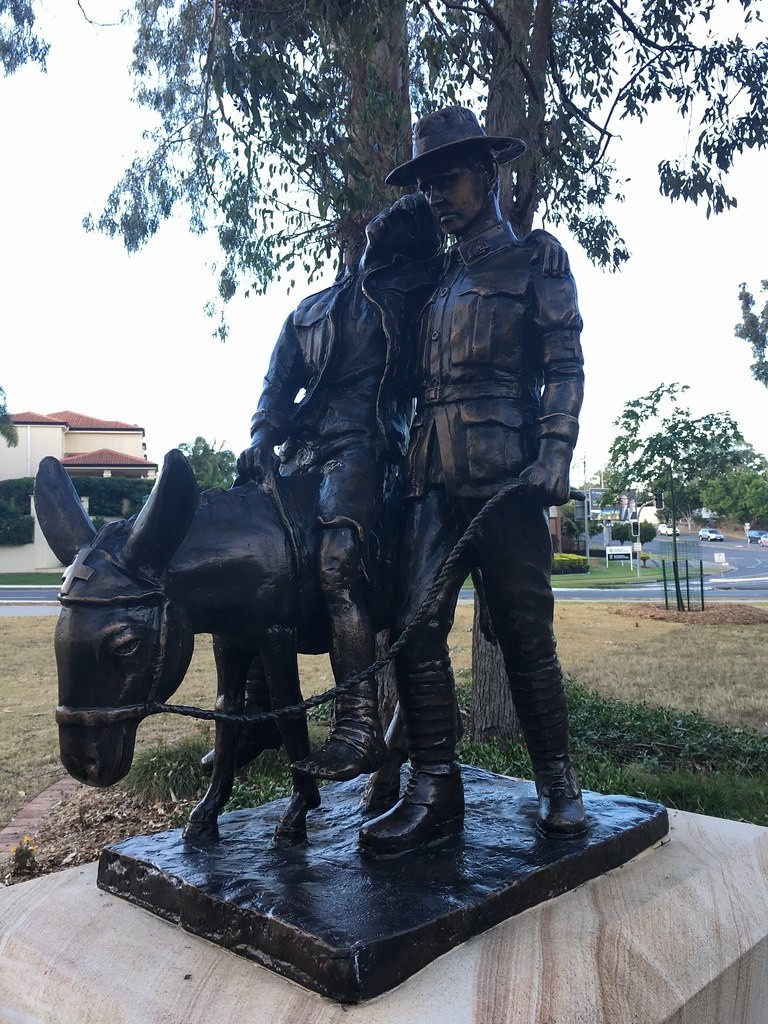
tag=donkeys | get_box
[32,447,415,854]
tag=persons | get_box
[359,107,587,863]
[201,194,570,782]
[619,492,636,520]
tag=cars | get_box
[658,524,680,537]
[747,530,768,545]
[760,534,768,547]
[698,529,724,542]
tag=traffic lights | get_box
[654,491,664,509]
[632,521,639,536]
